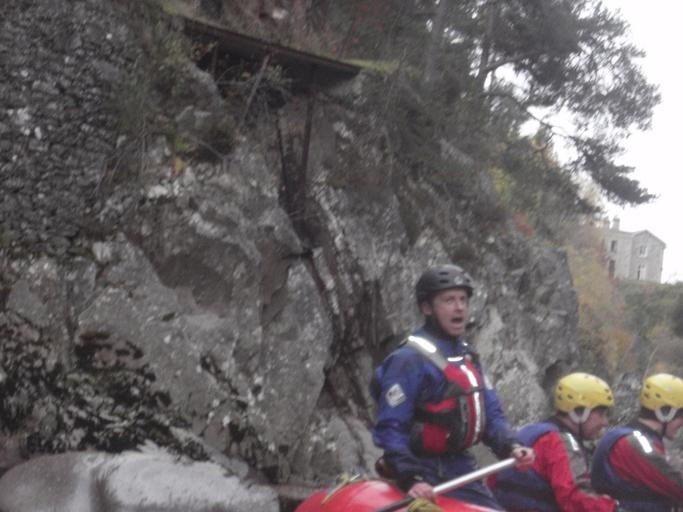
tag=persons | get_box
[370,264,535,512]
[590,373,683,512]
[493,372,620,512]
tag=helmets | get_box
[556,373,614,424]
[640,374,683,423]
[415,264,473,305]
